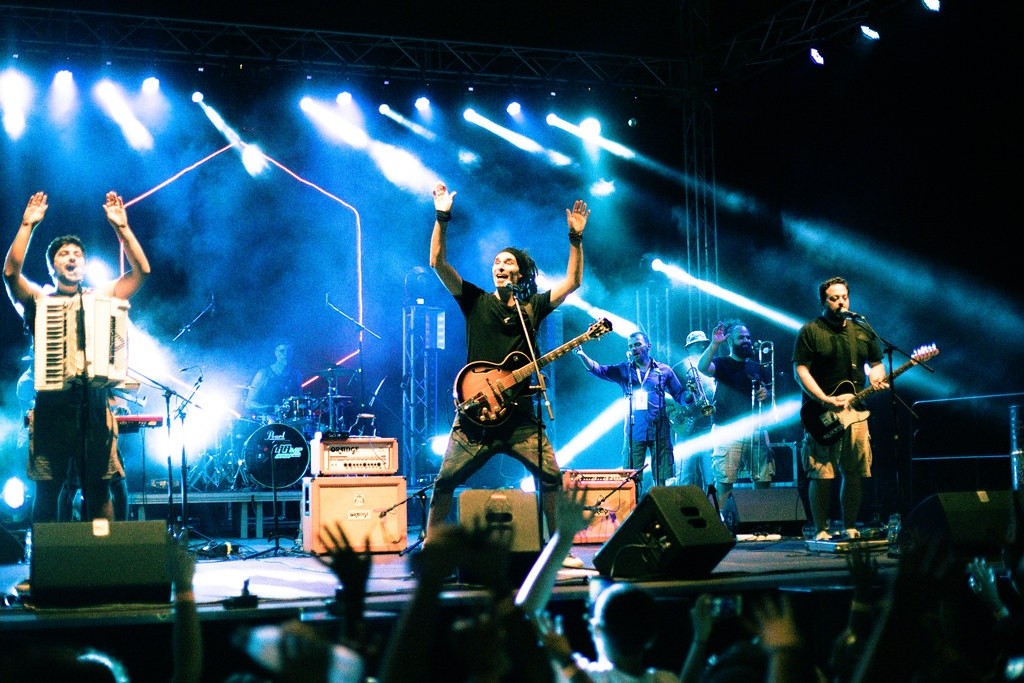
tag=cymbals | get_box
[234,385,259,390]
[322,395,352,399]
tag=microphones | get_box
[626,351,631,358]
[379,511,386,518]
[325,291,329,306]
[211,291,217,311]
[840,308,862,319]
[507,284,526,294]
[582,505,604,511]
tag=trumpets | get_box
[686,357,717,417]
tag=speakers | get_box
[302,476,408,552]
[559,468,636,543]
[895,489,1019,570]
[722,489,808,538]
[29,519,173,605]
[591,485,737,582]
[458,488,541,589]
[0,525,26,565]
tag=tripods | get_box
[174,416,249,546]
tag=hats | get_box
[684,330,711,350]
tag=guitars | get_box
[800,344,940,447]
[452,317,614,427]
[665,381,716,436]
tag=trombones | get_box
[750,340,784,481]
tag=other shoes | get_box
[812,529,833,541]
[15,579,31,592]
[561,552,584,569]
[842,528,861,539]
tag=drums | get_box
[283,396,313,422]
[241,423,310,489]
[230,418,257,444]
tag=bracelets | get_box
[568,233,583,241]
[436,210,452,222]
[175,591,195,602]
[994,606,1008,621]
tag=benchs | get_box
[121,485,473,541]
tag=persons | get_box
[0,483,1024,683]
[2,191,150,592]
[244,335,304,411]
[422,181,590,568]
[791,276,890,540]
[572,321,783,521]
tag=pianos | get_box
[24,414,162,520]
